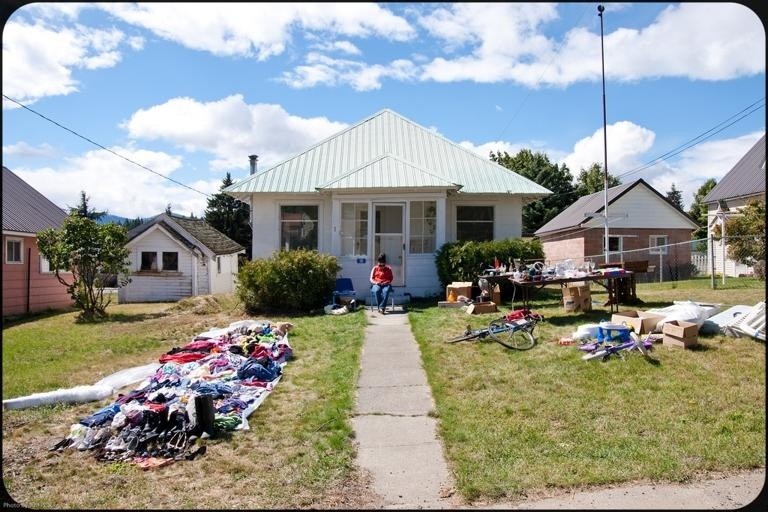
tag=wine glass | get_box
[577,261,596,274]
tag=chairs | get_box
[333,278,356,312]
[370,285,394,312]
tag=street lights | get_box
[716,210,727,289]
[593,3,611,267]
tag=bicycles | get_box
[445,306,542,352]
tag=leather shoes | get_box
[48,406,190,462]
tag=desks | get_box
[508,271,634,320]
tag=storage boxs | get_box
[663,321,698,348]
[446,282,501,315]
[611,310,664,335]
[561,285,592,312]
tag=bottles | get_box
[448,290,455,302]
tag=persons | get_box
[368,252,394,315]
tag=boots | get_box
[189,395,215,442]
